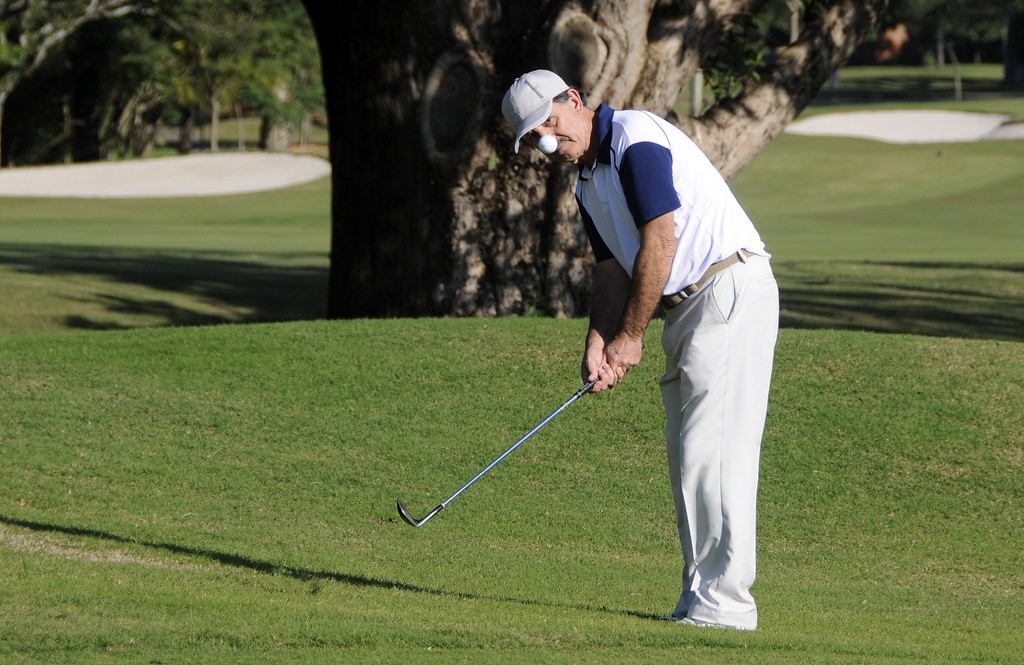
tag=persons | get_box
[502,70,779,631]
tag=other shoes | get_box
[659,612,735,629]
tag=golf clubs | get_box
[396,343,647,529]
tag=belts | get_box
[660,250,754,310]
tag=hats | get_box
[502,70,569,154]
